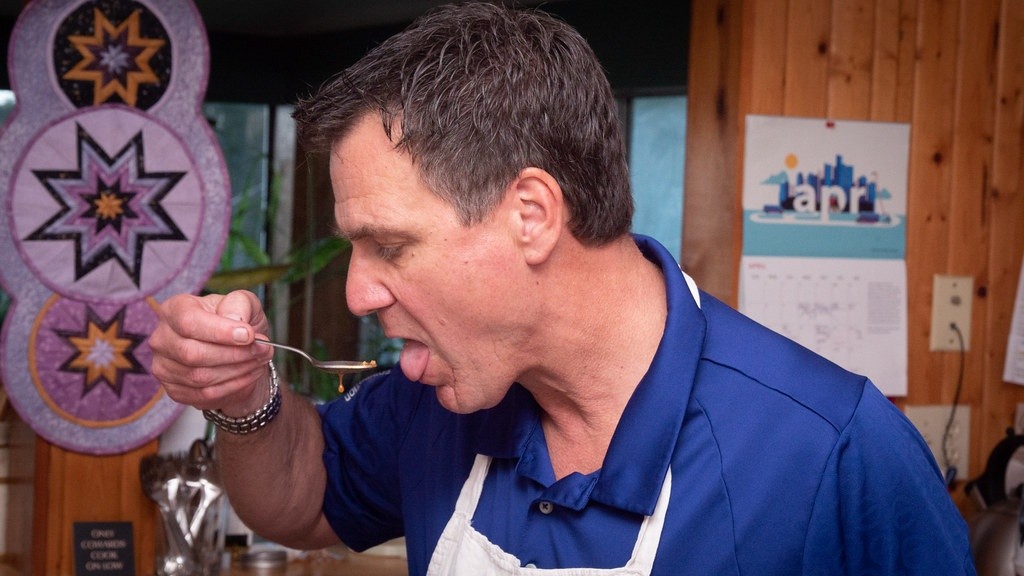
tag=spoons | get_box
[253,337,379,376]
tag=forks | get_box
[137,451,227,576]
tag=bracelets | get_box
[202,361,281,435]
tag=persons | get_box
[149,0,978,576]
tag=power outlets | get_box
[908,407,972,484]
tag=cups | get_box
[152,483,226,576]
